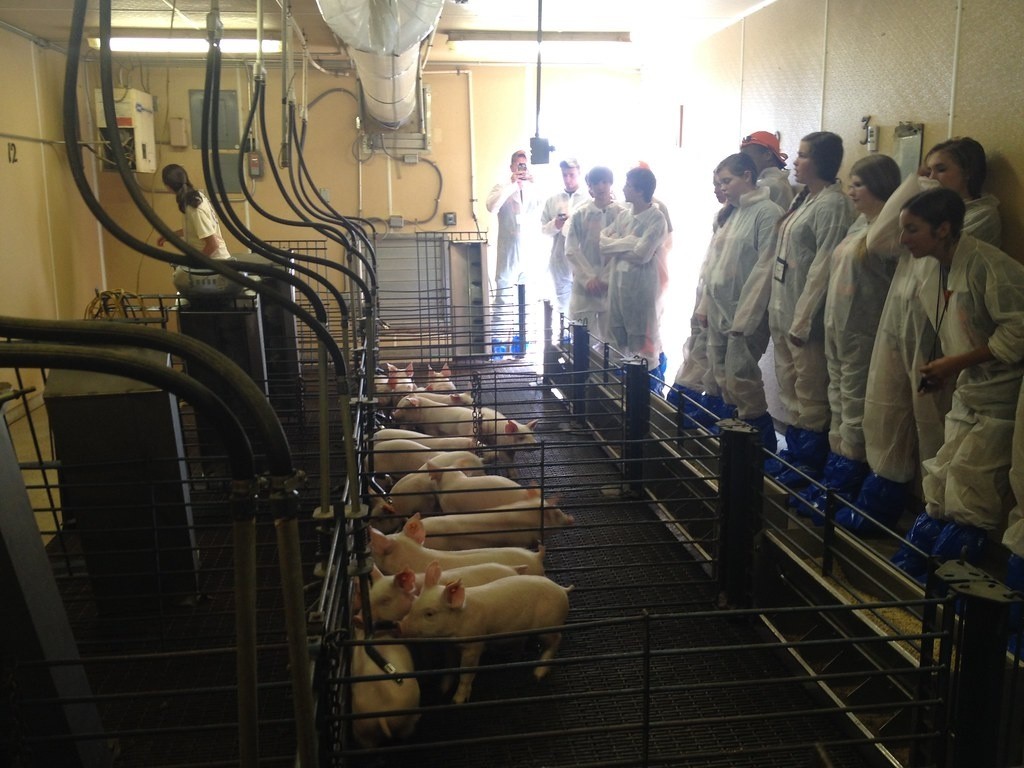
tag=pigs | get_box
[352,361,575,737]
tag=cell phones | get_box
[518,163,526,180]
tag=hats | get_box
[740,131,788,166]
[629,160,651,170]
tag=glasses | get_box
[742,136,761,142]
[847,182,866,190]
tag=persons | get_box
[765,131,854,487]
[158,164,230,259]
[670,131,794,453]
[565,166,626,358]
[837,136,1003,602]
[599,161,673,396]
[896,187,1024,660]
[540,157,593,344]
[486,150,547,361]
[789,155,900,528]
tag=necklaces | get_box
[916,261,951,393]
